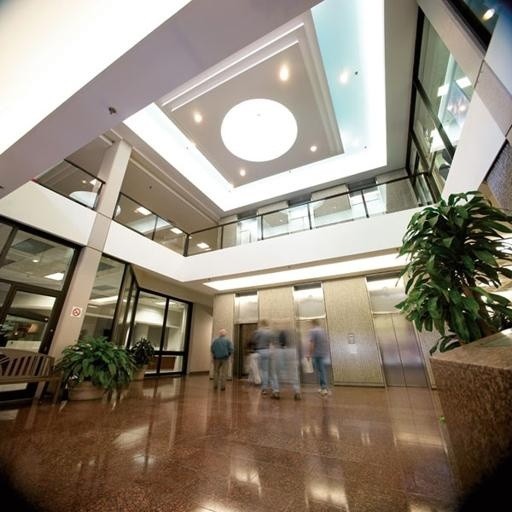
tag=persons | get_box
[211,329,234,391]
[246,318,332,400]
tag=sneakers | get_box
[261,388,328,401]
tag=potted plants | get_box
[53,334,155,401]
